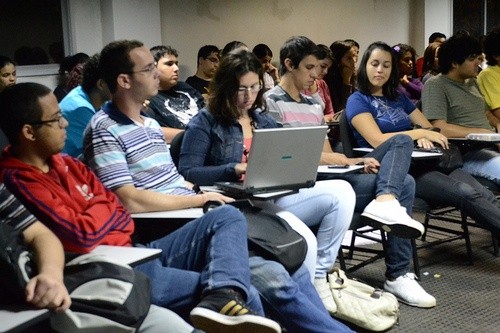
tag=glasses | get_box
[205,57,219,63]
[239,79,263,93]
[121,61,157,74]
[26,109,63,125]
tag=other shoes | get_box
[314,278,337,313]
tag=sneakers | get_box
[190,290,281,333]
[360,197,425,239]
[383,272,436,308]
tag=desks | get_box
[317,165,364,177]
[352,147,444,161]
[89,245,163,268]
[448,138,500,147]
[199,185,293,202]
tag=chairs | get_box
[170,99,490,280]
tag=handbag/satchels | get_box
[409,142,463,172]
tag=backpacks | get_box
[326,267,400,331]
[0,223,151,333]
[203,198,308,276]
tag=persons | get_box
[302,38,360,147]
[58,54,113,160]
[0,82,281,333]
[253,43,280,89]
[263,35,437,307]
[0,179,206,333]
[186,40,248,104]
[53,52,90,103]
[84,39,355,333]
[0,57,16,90]
[179,46,356,313]
[140,46,204,144]
[393,32,500,199]
[345,41,500,231]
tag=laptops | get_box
[214,125,329,195]
